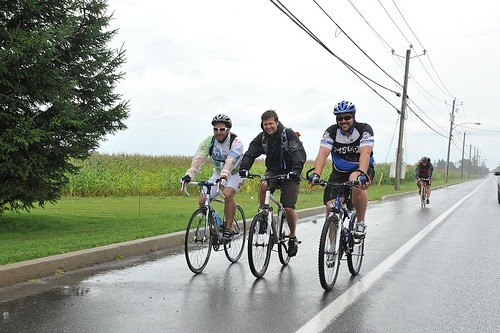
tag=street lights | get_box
[445,122,481,183]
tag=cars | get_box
[494,167,500,204]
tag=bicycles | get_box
[416,177,430,211]
[240,171,302,278]
[181,179,246,273]
[306,167,371,291]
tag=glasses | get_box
[214,128,229,131]
[336,116,353,121]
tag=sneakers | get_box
[259,218,267,233]
[222,229,234,241]
[354,224,365,239]
[327,252,336,267]
[288,236,298,256]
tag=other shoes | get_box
[426,199,429,204]
[418,189,421,195]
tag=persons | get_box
[182,114,247,244]
[308,101,376,268]
[415,157,433,204]
[239,110,307,257]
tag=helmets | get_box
[333,101,356,115]
[212,114,231,128]
[422,157,428,161]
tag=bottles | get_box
[344,228,351,247]
[214,210,222,224]
